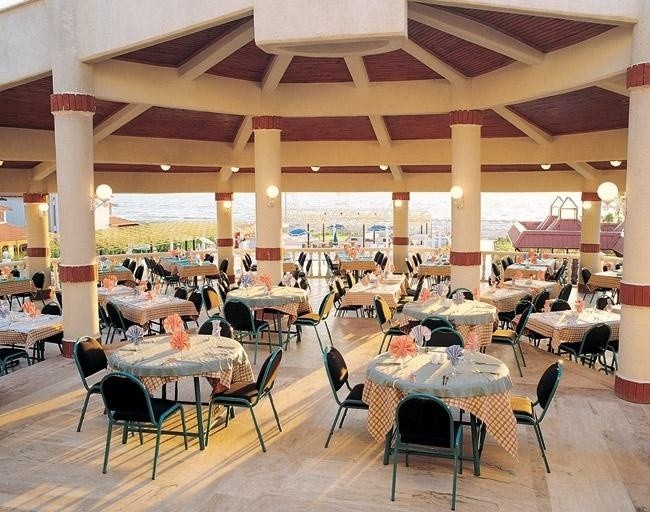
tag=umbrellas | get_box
[366,222,393,238]
[326,223,347,235]
[289,228,308,238]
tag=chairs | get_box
[382,393,465,509]
[205,346,282,453]
[0,250,625,374]
[478,361,563,474]
[101,373,187,479]
[324,347,370,449]
[73,335,107,432]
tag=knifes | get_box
[376,346,502,389]
[119,334,238,367]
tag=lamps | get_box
[223,200,231,215]
[265,185,279,209]
[596,181,628,217]
[38,203,49,216]
[451,185,464,210]
[89,184,114,216]
